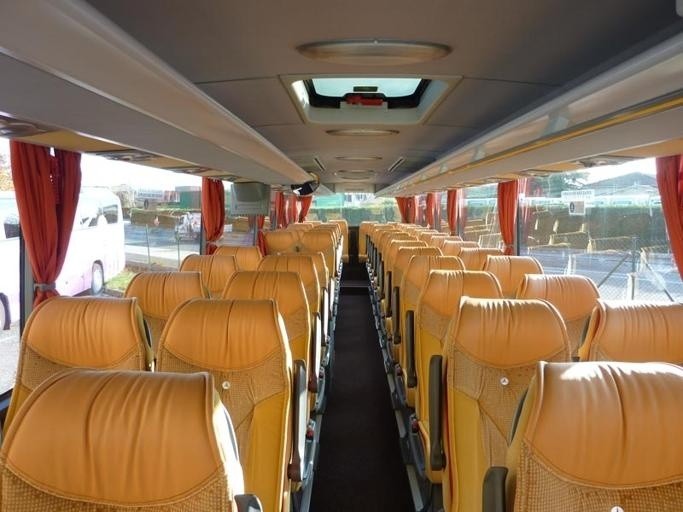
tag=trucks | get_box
[175,211,234,243]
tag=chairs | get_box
[178,215,351,415]
[476,357,681,508]
[0,365,264,510]
[217,267,315,489]
[575,296,681,365]
[423,293,575,510]
[153,293,313,510]
[355,217,547,441]
[405,267,511,492]
[2,294,153,443]
[120,268,209,360]
[515,270,602,357]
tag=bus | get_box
[0,187,124,333]
